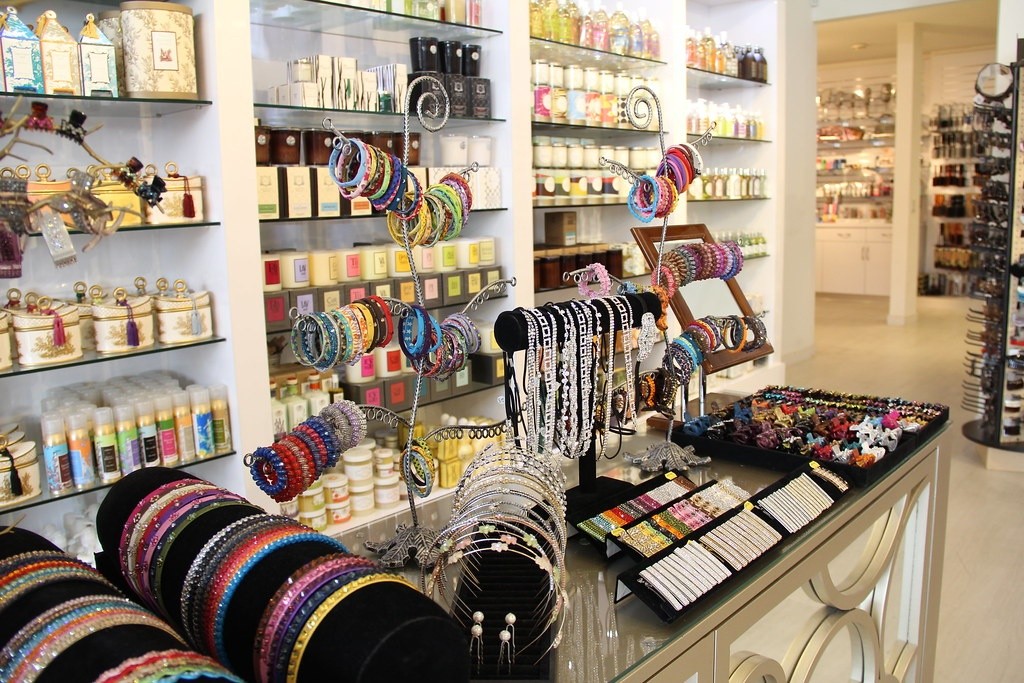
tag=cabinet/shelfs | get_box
[685,66,772,262]
[531,33,669,294]
[0,0,236,520]
[248,0,512,539]
[923,128,987,281]
[814,134,894,297]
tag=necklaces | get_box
[501,297,639,462]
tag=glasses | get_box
[964,100,1014,299]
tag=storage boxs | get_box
[266,55,492,120]
[338,352,504,418]
[264,266,503,334]
[545,211,577,247]
[257,164,499,220]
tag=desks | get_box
[331,392,954,683]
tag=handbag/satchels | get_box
[0,162,213,371]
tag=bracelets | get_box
[329,138,473,249]
[290,296,484,381]
[670,313,768,383]
[651,238,744,296]
[250,400,368,502]
[629,140,705,221]
[395,437,435,498]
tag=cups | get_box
[462,44,481,77]
[437,40,464,75]
[375,346,402,378]
[468,135,493,167]
[262,237,498,293]
[346,348,376,384]
[480,327,503,353]
[409,36,439,73]
[531,59,661,132]
[438,132,467,167]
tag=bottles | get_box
[270,371,344,442]
[41,371,233,497]
[530,0,660,60]
[686,24,767,260]
